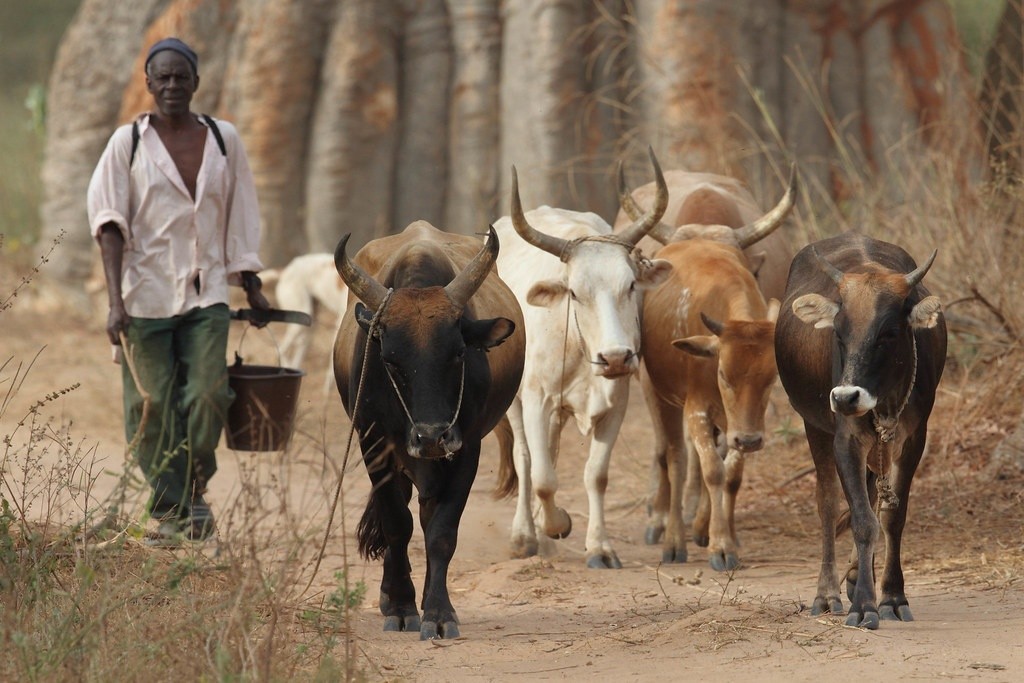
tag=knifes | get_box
[230,308,311,326]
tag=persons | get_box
[87,40,270,547]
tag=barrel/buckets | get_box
[224,323,305,452]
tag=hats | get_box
[145,38,198,75]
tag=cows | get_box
[332,143,949,643]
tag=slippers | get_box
[179,498,215,542]
[146,519,180,550]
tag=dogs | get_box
[254,251,348,400]
